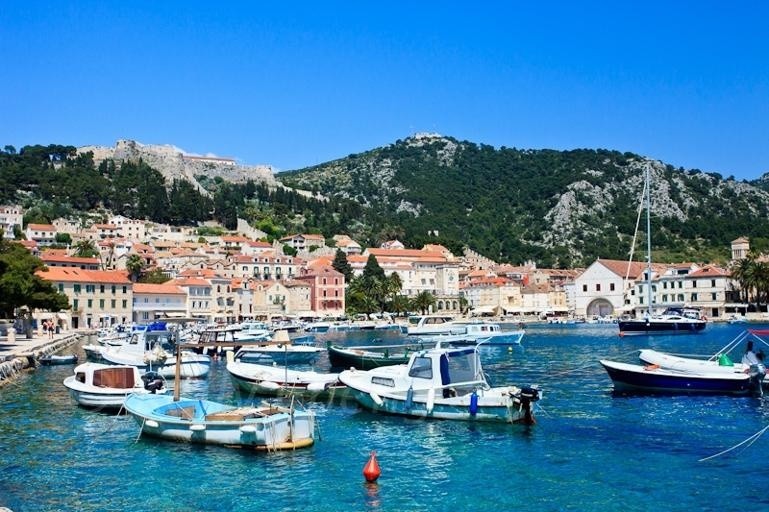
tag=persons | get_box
[47,319,54,339]
[43,322,48,331]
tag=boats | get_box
[596,329,769,393]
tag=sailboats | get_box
[619,165,705,337]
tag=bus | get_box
[677,309,703,320]
[547,316,564,324]
